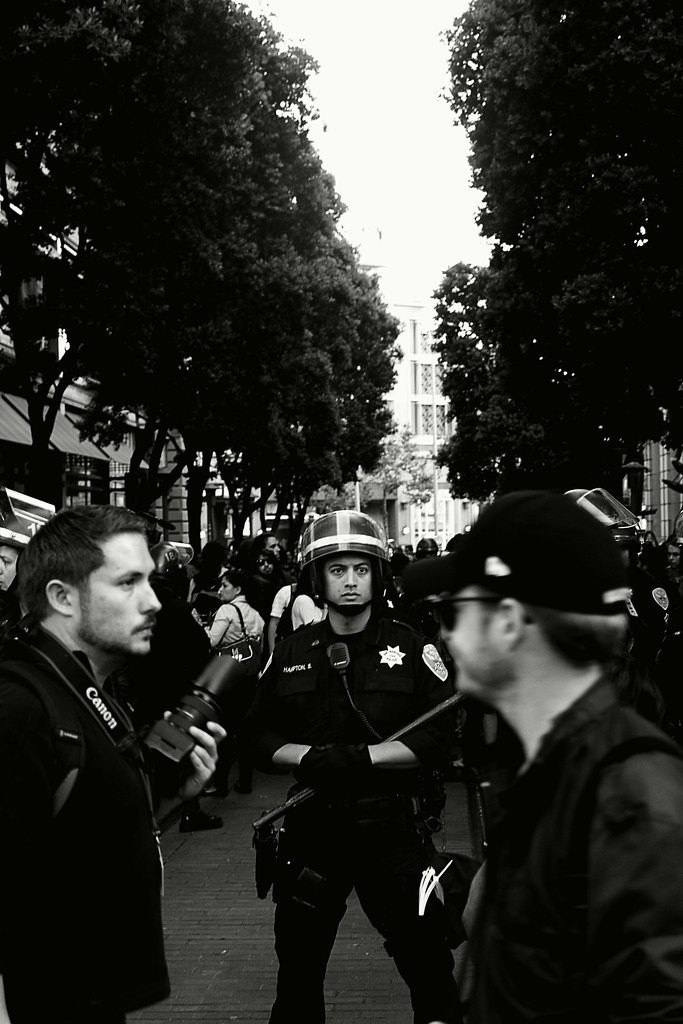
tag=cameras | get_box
[144,654,246,779]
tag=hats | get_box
[401,490,634,616]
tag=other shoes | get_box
[200,781,228,797]
[234,782,252,794]
[179,808,223,832]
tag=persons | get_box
[402,491,683,1024]
[237,512,465,1024]
[0,496,331,832]
[0,505,227,1024]
[383,532,525,783]
[569,487,683,728]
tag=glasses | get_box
[433,596,534,631]
[256,558,273,566]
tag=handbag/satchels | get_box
[209,603,262,677]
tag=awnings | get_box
[0,392,149,468]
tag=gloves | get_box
[294,742,373,782]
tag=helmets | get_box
[563,487,640,547]
[297,510,391,570]
[417,538,438,558]
[149,541,194,583]
[0,487,56,550]
